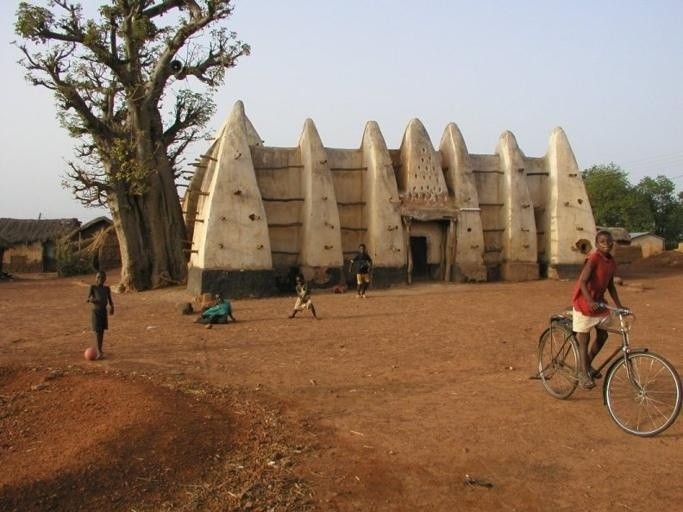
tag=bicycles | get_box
[528,302,683,437]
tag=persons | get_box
[86,270,113,360]
[287,273,319,320]
[193,293,237,330]
[570,231,631,390]
[348,243,373,297]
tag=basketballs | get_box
[85,347,97,359]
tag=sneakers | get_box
[577,365,603,389]
